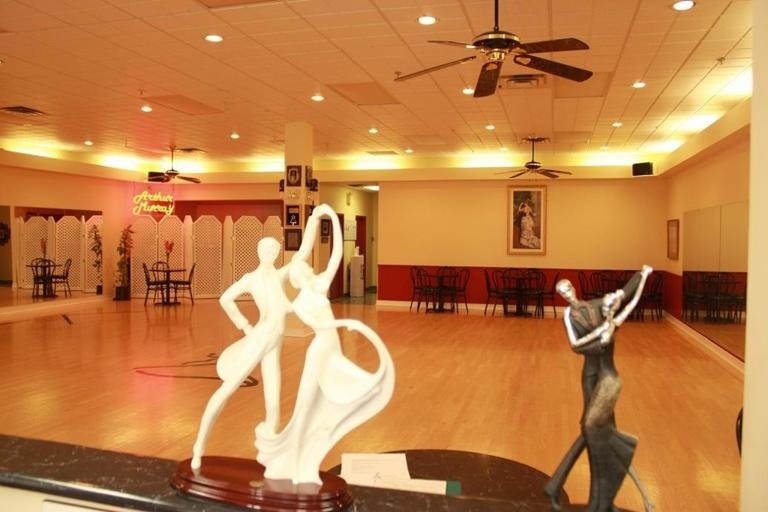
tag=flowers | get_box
[163,241,174,267]
[39,237,48,259]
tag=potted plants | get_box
[112,224,136,300]
[88,224,103,296]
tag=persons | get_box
[514,197,535,245]
[542,265,648,511]
[253,203,397,484]
[190,206,326,471]
[517,201,541,249]
[562,264,656,512]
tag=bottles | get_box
[290,215,297,225]
[354,246,360,255]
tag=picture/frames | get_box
[667,219,680,260]
[285,204,332,251]
[506,184,548,256]
[286,164,313,187]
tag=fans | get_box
[147,143,202,184]
[394,0,594,99]
[495,136,572,179]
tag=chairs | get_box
[483,265,560,319]
[576,270,665,322]
[142,261,199,307]
[409,266,471,315]
[683,269,747,330]
[25,257,72,298]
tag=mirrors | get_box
[680,199,748,362]
[0,204,103,322]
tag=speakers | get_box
[148,171,164,182]
[632,162,653,175]
